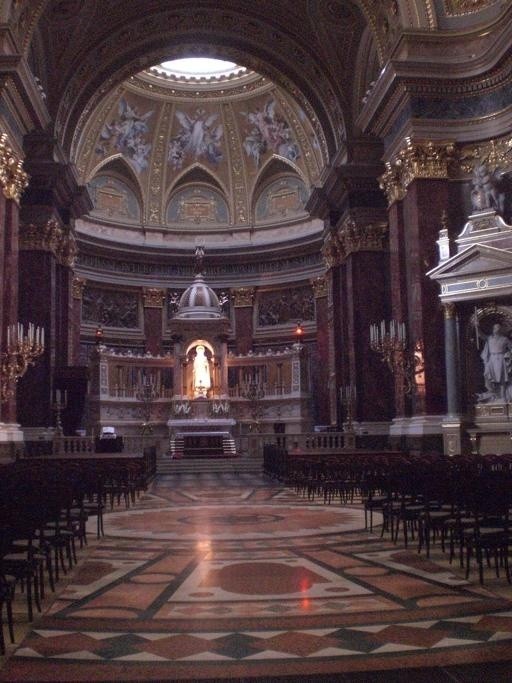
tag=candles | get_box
[367,317,411,344]
[0,320,45,353]
[340,384,359,399]
[46,387,69,406]
[135,369,162,393]
[237,371,267,388]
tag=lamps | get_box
[94,323,104,347]
[294,318,305,343]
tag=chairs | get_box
[261,439,512,596]
[1,444,158,661]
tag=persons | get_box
[477,164,499,208]
[193,344,212,398]
[193,243,207,278]
[474,322,511,403]
[217,292,229,312]
[168,290,180,314]
